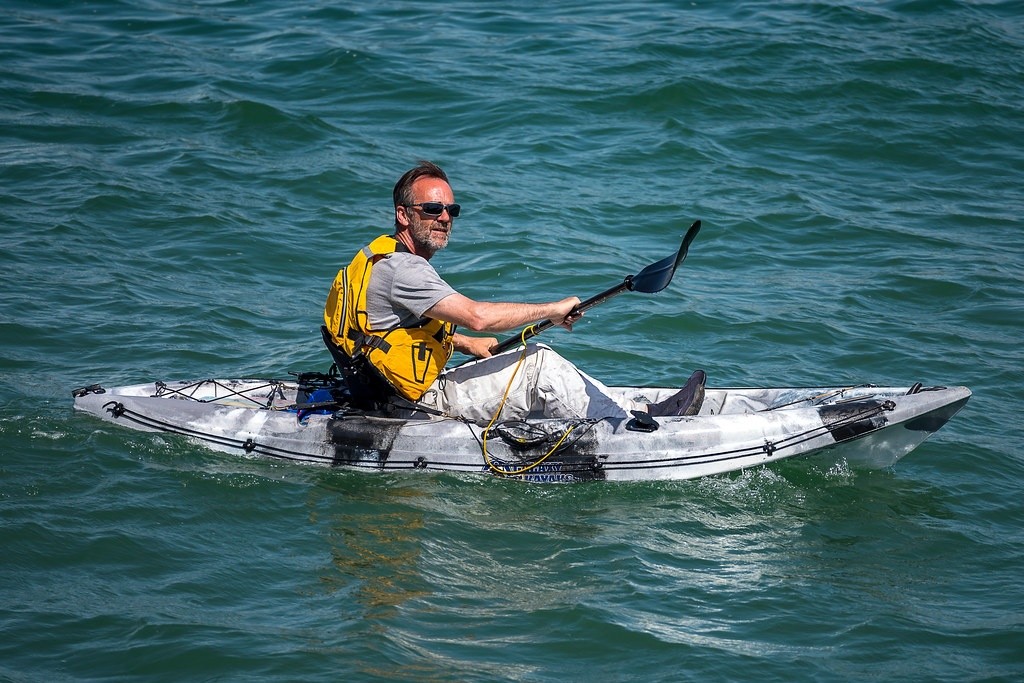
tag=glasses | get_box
[401,202,461,218]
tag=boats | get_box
[73,376,975,484]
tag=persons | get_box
[321,161,709,433]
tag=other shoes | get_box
[647,370,706,416]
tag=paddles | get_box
[445,220,703,370]
